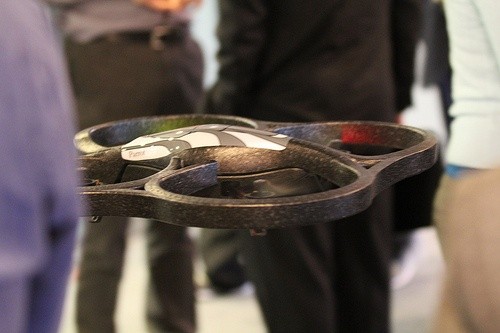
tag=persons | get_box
[207,1,454,332]
[52,1,204,333]
